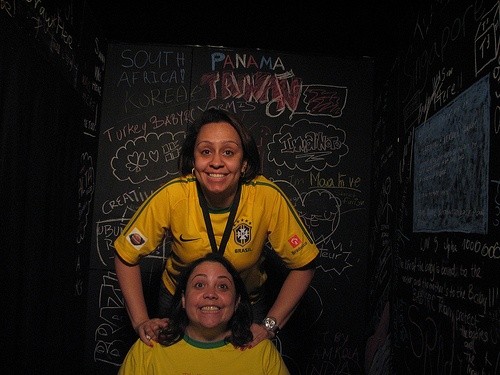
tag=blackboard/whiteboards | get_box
[0,0,500,375]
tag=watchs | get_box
[259,316,281,337]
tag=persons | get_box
[117,252,291,375]
[112,109,322,347]
